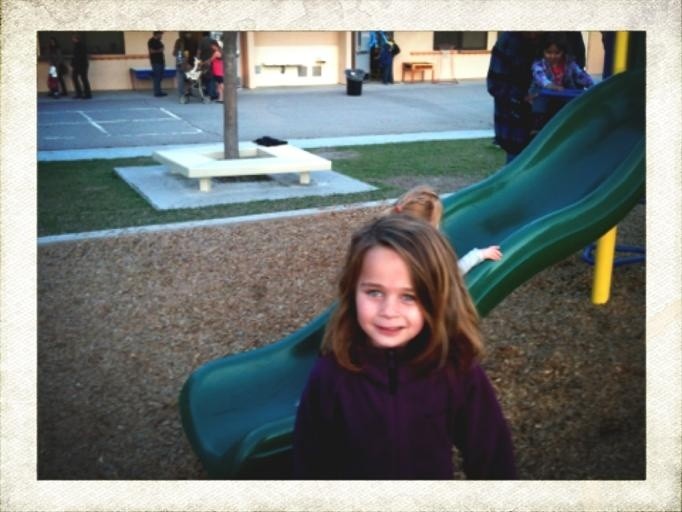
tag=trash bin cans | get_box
[346,70,365,95]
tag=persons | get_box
[196,40,228,105]
[68,34,93,100]
[171,32,196,103]
[377,32,398,84]
[189,32,216,99]
[147,31,169,97]
[522,37,599,142]
[46,37,70,98]
[279,214,523,480]
[380,186,504,280]
[486,31,586,168]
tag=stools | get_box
[402,62,437,84]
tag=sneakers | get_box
[47,88,92,101]
[154,89,169,98]
[180,90,226,105]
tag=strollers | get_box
[180,58,212,104]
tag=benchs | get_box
[129,66,185,93]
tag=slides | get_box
[179,65,648,480]
